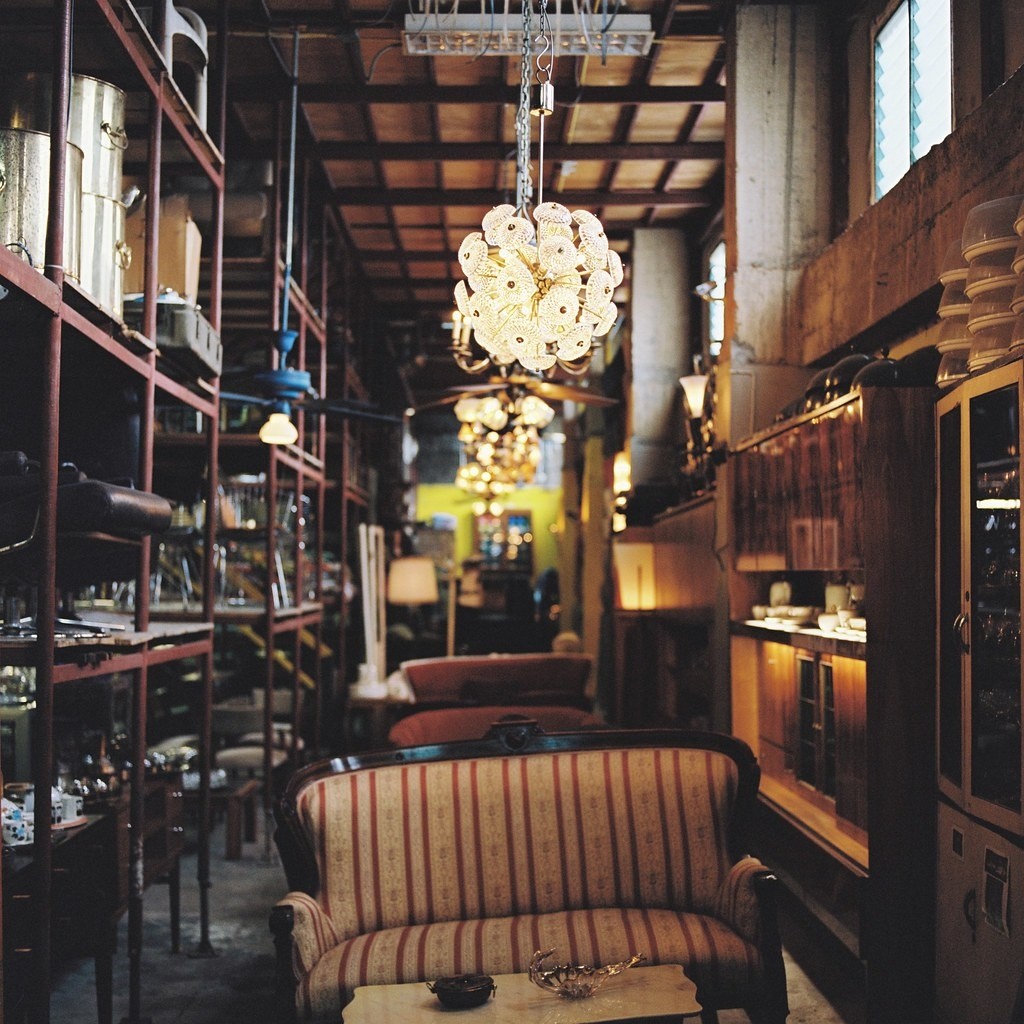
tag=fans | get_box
[415,371,617,410]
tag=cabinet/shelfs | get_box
[0,0,377,1024]
[729,386,932,1024]
[932,351,1024,1024]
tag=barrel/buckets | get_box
[1,67,127,202]
[82,185,139,320]
[0,122,83,287]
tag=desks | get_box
[341,965,702,1024]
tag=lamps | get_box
[389,558,440,660]
[253,27,312,445]
[453,15,624,376]
[453,388,556,515]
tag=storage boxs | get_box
[123,176,202,306]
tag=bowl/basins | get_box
[752,604,866,637]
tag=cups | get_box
[1,782,84,846]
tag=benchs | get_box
[384,695,602,748]
[267,729,793,1024]
[402,653,594,702]
